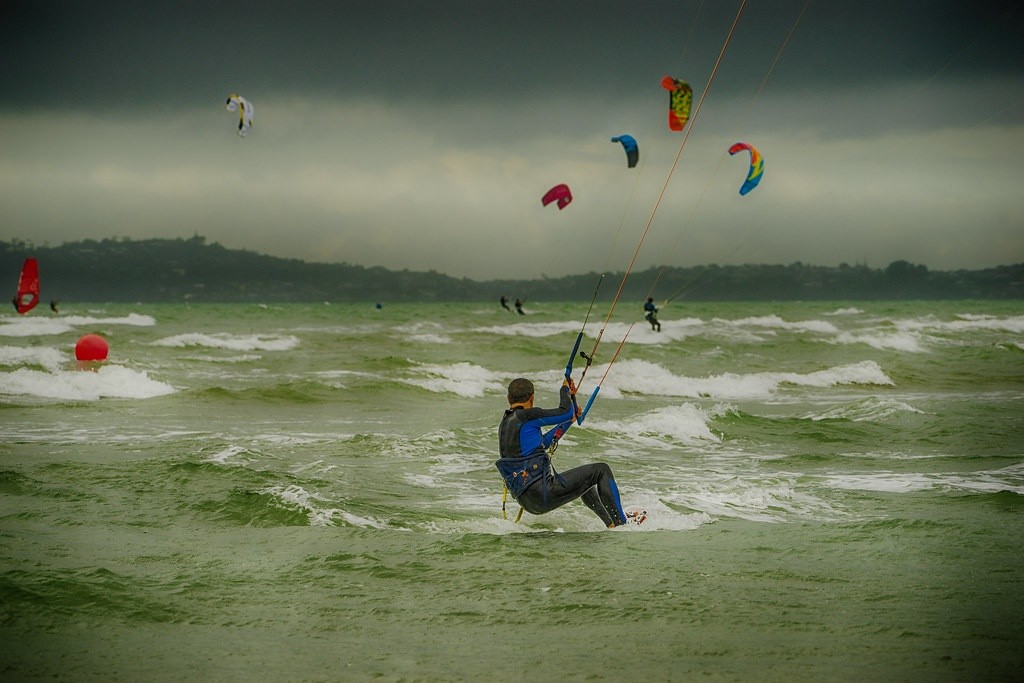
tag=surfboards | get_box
[623,506,647,525]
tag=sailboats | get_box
[15,256,41,316]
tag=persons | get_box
[12,296,19,311]
[501,296,510,311]
[50,299,58,312]
[644,297,660,332]
[495,378,647,528]
[515,299,525,315]
